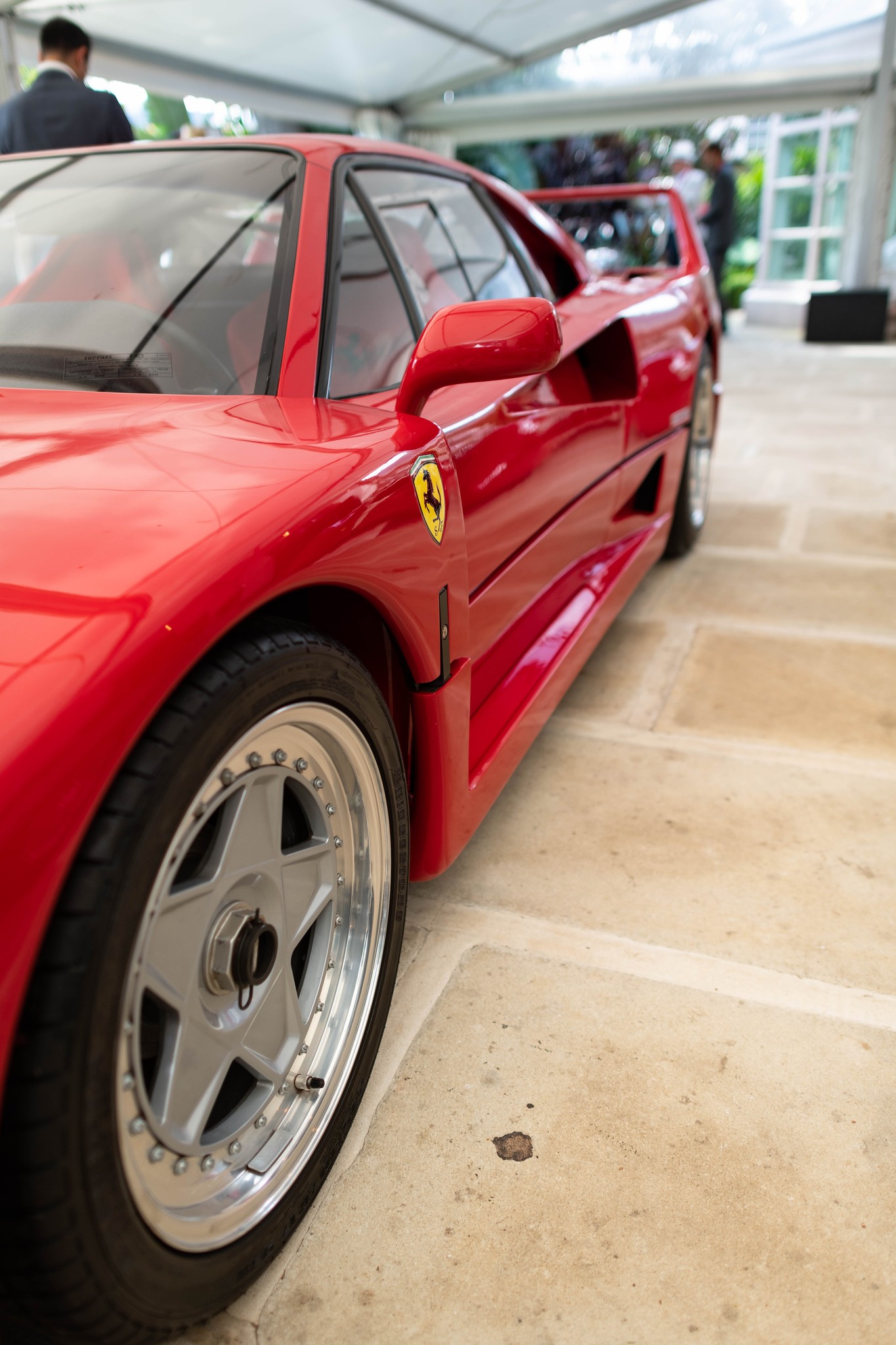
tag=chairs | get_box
[0,227,185,396]
[227,216,468,401]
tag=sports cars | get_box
[0,129,729,1344]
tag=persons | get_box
[0,17,134,284]
[651,132,736,333]
